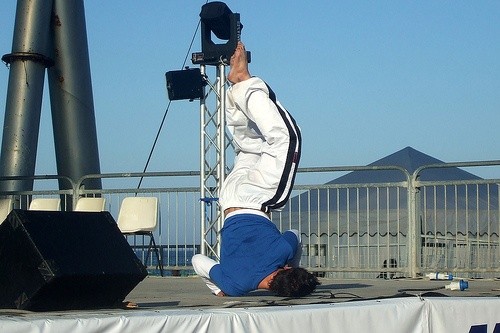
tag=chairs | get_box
[73,197,106,212]
[0,199,13,225]
[30,198,61,210]
[116,197,163,276]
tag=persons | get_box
[191,42,320,297]
[377,259,397,279]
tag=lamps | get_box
[200,1,243,64]
[165,67,203,100]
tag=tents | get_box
[269,146,500,279]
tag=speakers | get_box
[165,69,204,100]
[0,209,148,313]
